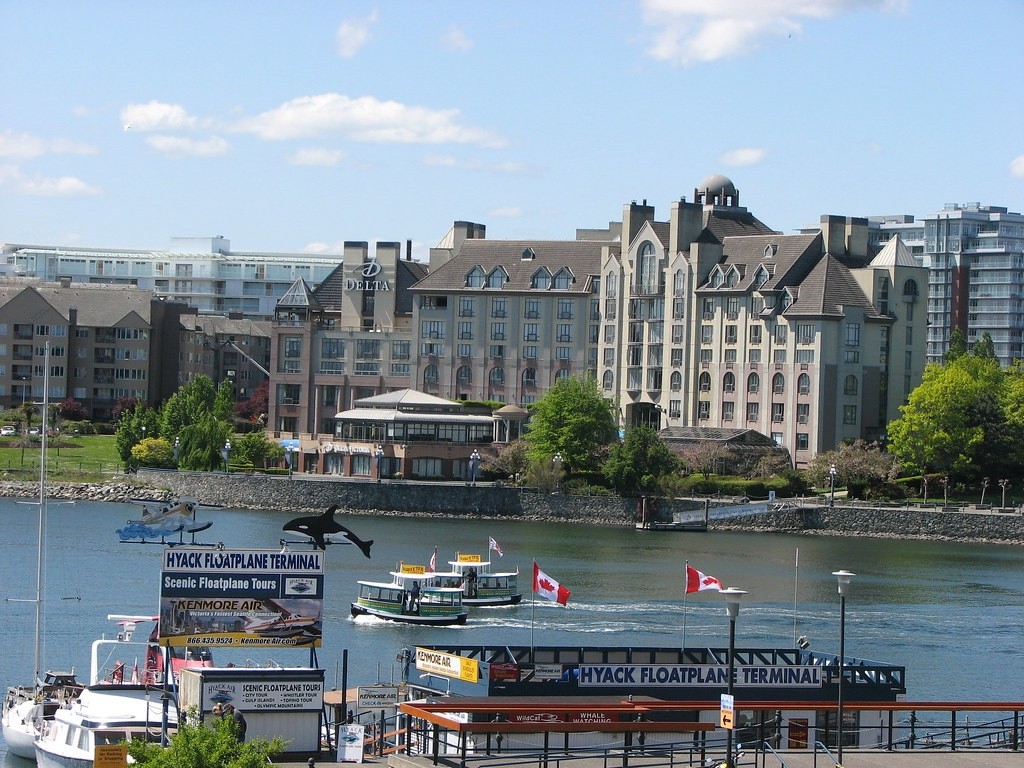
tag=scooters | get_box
[706,743,745,768]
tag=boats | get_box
[422,551,524,607]
[0,340,217,768]
[349,560,470,626]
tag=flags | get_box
[429,545,438,572]
[684,565,724,594]
[489,536,504,557]
[532,560,570,606]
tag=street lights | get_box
[469,448,481,486]
[225,439,231,474]
[552,452,565,494]
[374,444,384,483]
[718,586,749,768]
[828,463,835,506]
[832,570,856,768]
[141,427,146,445]
[287,441,294,479]
[175,436,180,470]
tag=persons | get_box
[397,568,503,615]
[212,702,247,743]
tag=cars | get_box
[24,426,40,437]
[1,425,17,436]
